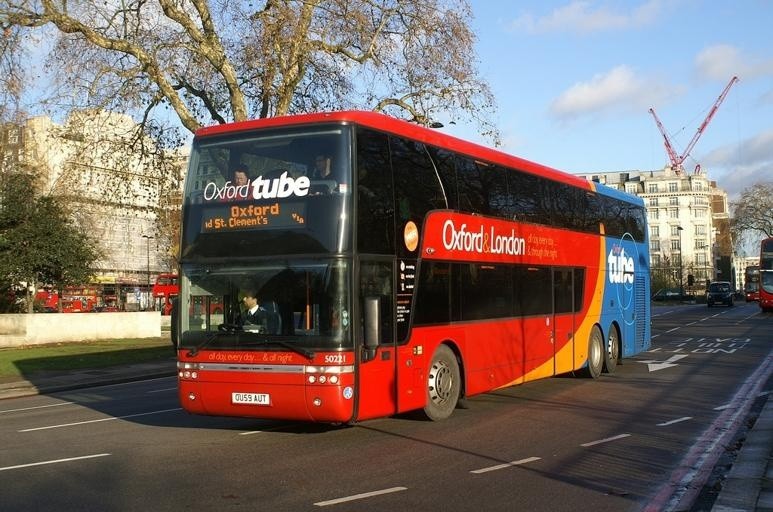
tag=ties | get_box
[248,311,252,317]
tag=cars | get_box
[706,281,735,308]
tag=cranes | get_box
[647,76,741,172]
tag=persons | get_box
[218,164,254,202]
[309,152,343,195]
[235,287,267,334]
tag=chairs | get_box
[262,169,288,194]
[253,299,281,337]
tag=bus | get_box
[34,285,119,313]
[744,238,773,313]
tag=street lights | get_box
[677,227,683,287]
[143,235,153,292]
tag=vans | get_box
[652,287,686,300]
